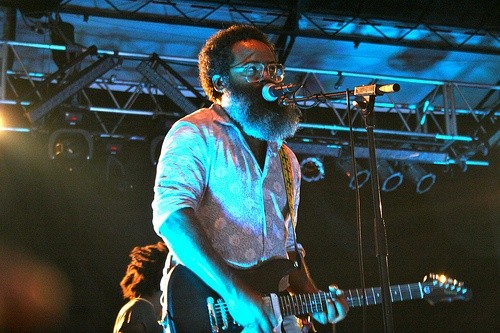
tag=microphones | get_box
[262,81,300,103]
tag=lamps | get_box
[0,114,491,197]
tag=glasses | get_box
[228,63,285,83]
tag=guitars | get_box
[161,263,472,332]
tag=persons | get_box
[110,242,171,332]
[152,21,356,333]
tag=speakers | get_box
[52,21,74,65]
